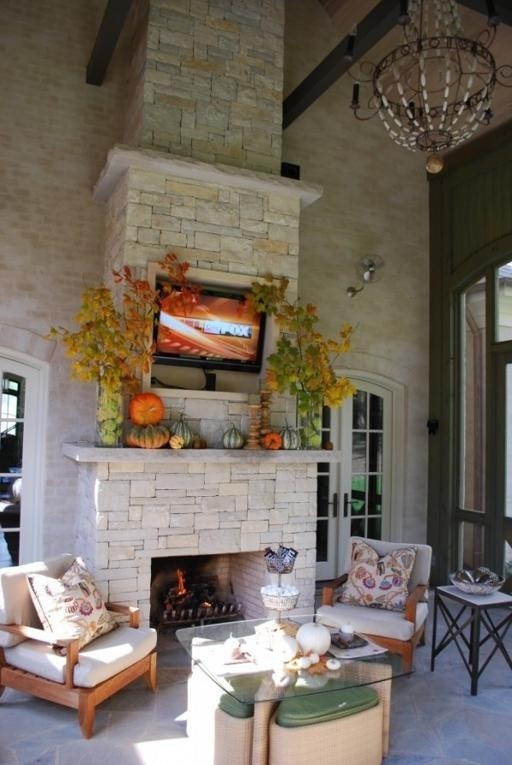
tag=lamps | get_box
[346,254,385,298]
[342,0,512,174]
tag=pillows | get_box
[26,557,120,656]
[335,538,418,612]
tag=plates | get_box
[327,633,389,658]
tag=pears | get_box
[324,442,333,449]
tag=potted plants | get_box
[44,252,201,449]
[243,272,359,451]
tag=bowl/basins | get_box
[448,570,505,595]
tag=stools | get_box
[267,676,384,765]
[185,671,262,765]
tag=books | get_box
[191,634,299,677]
[327,627,389,659]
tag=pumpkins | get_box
[280,428,298,449]
[193,435,207,448]
[270,669,329,689]
[223,423,244,449]
[170,413,194,447]
[170,435,183,449]
[270,620,341,676]
[263,433,281,450]
[124,425,169,448]
[127,393,164,426]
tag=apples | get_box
[100,400,124,445]
[301,418,321,447]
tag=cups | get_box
[338,622,356,644]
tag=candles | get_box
[257,377,268,391]
[247,393,262,407]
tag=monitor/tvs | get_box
[151,272,267,374]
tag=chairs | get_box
[0,553,157,740]
[316,536,432,679]
